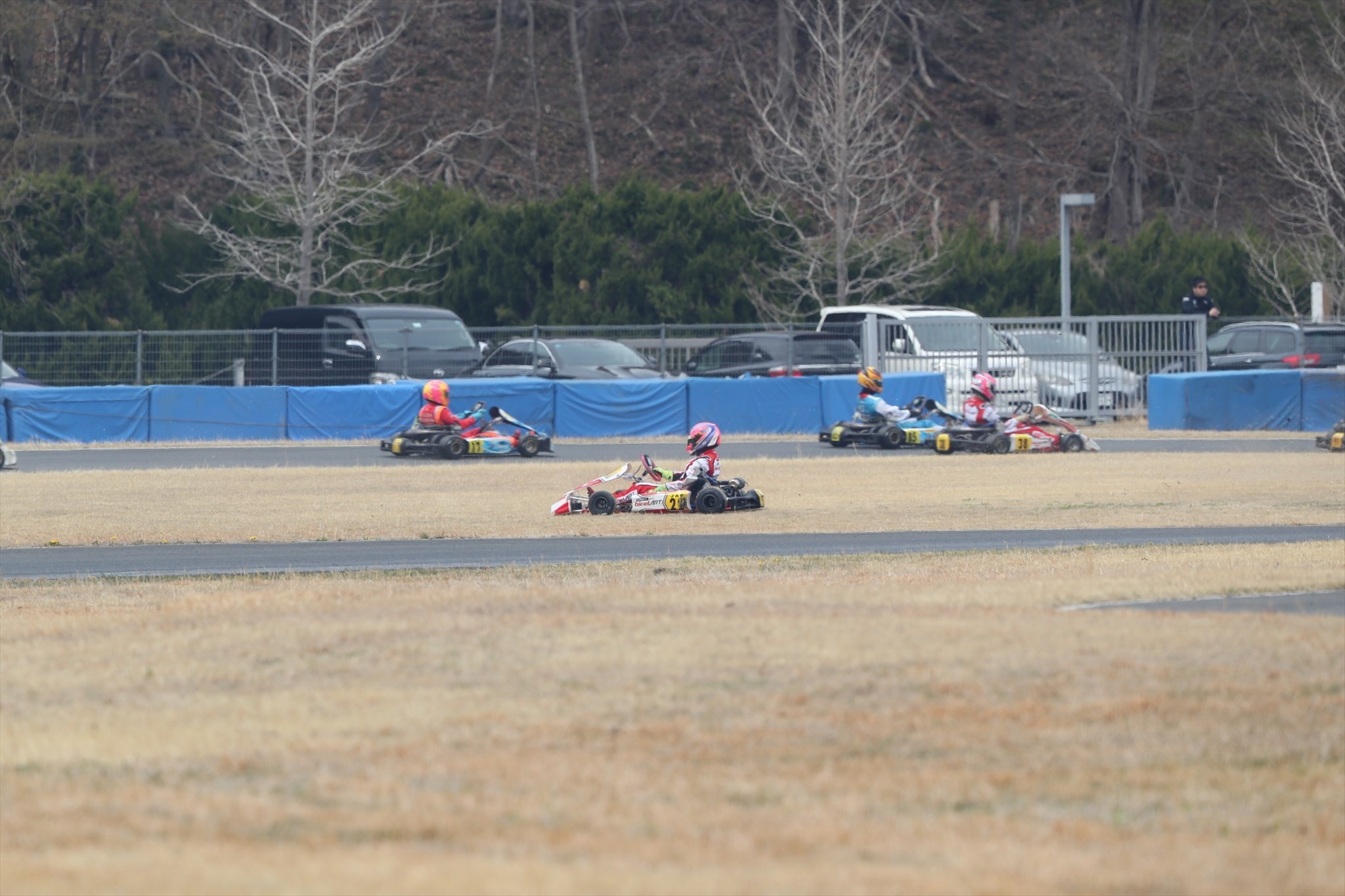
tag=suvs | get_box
[669,330,863,380]
[816,304,1038,420]
[1156,318,1345,376]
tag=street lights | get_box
[1060,193,1098,331]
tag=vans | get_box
[248,302,485,386]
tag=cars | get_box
[997,328,1145,419]
[453,335,678,380]
[0,360,45,387]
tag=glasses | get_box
[1197,285,1207,289]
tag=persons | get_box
[1182,276,1220,373]
[855,366,965,429]
[587,423,720,499]
[420,379,500,441]
[964,372,1059,438]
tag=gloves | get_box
[469,408,487,423]
[908,407,923,418]
[457,410,473,420]
[652,467,673,482]
[899,404,910,410]
[646,483,669,493]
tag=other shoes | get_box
[587,486,594,495]
[528,431,534,435]
[513,429,520,440]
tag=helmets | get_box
[971,372,996,403]
[423,379,449,406]
[686,422,721,456]
[857,366,884,394]
